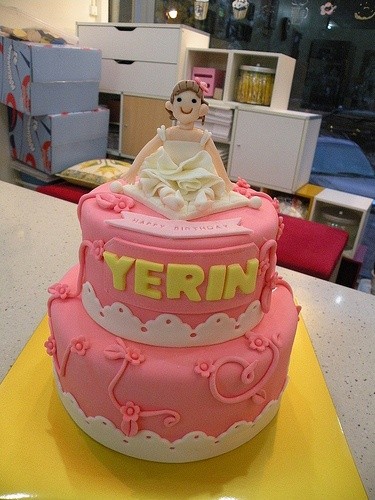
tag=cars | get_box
[309,136,375,210]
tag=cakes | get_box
[44,76,303,463]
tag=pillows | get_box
[55,159,133,187]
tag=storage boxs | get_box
[344,245,368,286]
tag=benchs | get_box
[37,170,350,279]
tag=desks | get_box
[2,181,374,499]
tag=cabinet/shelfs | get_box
[1,21,373,260]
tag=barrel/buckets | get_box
[236,64,275,106]
